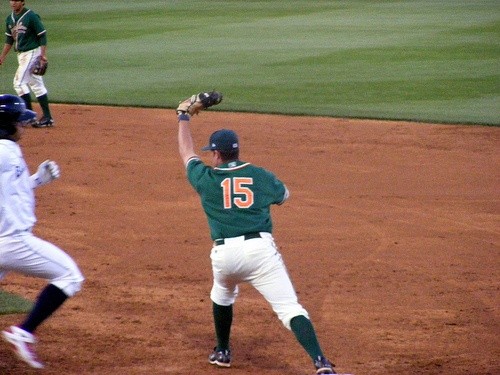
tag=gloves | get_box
[29,159,61,189]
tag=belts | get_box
[19,47,36,52]
[215,231,262,246]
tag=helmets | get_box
[0,94,37,121]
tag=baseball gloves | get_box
[176,91,223,117]
[29,56,48,76]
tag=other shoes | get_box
[208,346,232,367]
[313,356,336,375]
[31,115,55,128]
[26,116,37,124]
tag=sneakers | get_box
[1,322,44,369]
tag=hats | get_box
[201,129,238,150]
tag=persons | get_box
[176,91,338,375]
[0,0,56,128]
[0,94,85,369]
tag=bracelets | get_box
[177,115,189,122]
